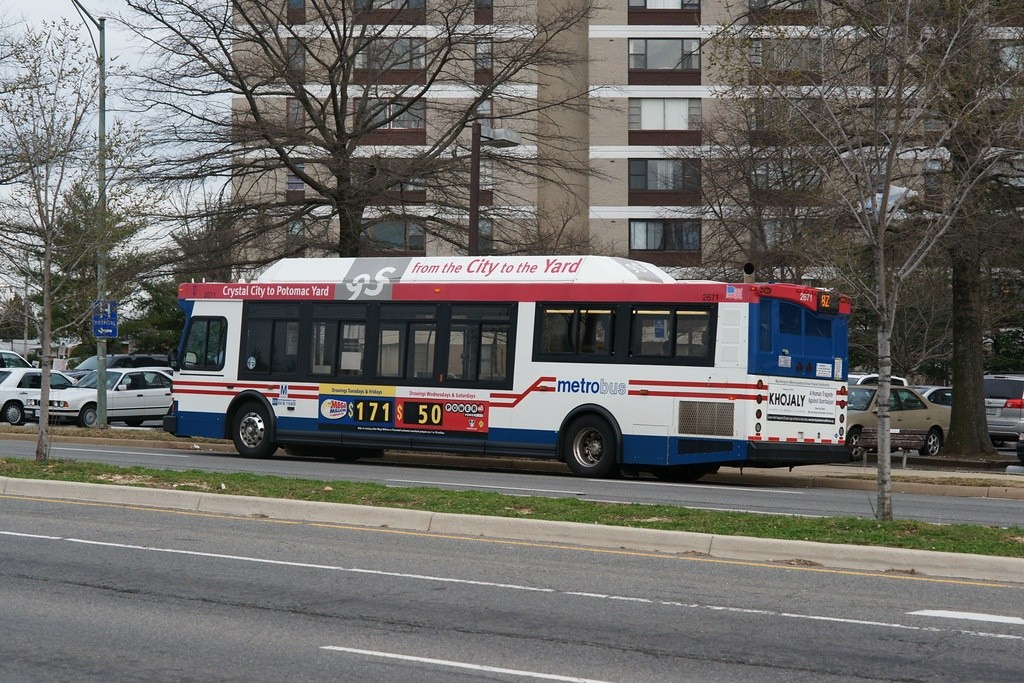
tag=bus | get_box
[161,256,851,479]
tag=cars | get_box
[897,384,953,405]
[848,372,907,389]
[0,367,78,425]
[847,386,951,457]
[26,368,173,427]
[0,350,169,381]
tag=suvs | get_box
[982,375,1024,446]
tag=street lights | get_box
[469,139,521,252]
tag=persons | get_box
[208,331,223,366]
